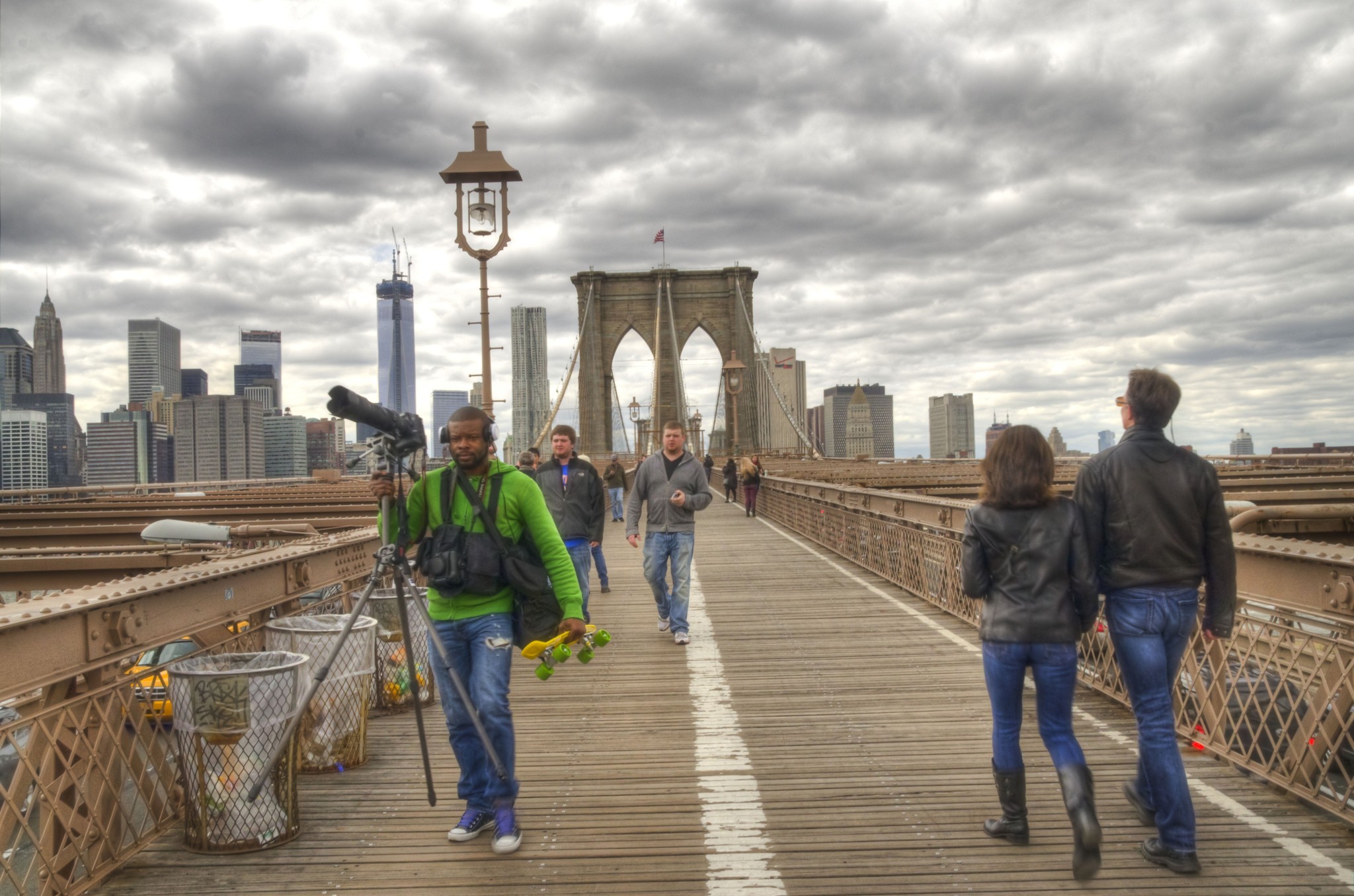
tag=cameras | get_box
[437,417,500,444]
[672,493,678,498]
[326,386,426,453]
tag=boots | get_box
[1056,765,1103,881]
[983,758,1030,846]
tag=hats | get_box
[611,454,617,461]
[577,454,591,464]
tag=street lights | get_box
[437,121,522,461]
[693,410,703,457]
[627,397,642,464]
[721,351,746,471]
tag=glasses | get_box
[1116,396,1135,407]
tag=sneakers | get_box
[674,631,690,645]
[490,809,523,854]
[657,615,670,631]
[448,807,498,841]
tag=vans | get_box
[123,622,250,731]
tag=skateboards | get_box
[519,625,606,680]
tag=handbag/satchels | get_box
[501,546,563,651]
[722,478,734,487]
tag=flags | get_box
[653,230,664,244]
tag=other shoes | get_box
[752,511,756,517]
[725,500,728,503]
[612,518,618,522]
[1123,780,1156,824]
[746,512,750,517]
[732,499,736,502]
[619,517,624,522]
[1142,836,1202,873]
[601,586,611,593]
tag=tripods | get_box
[248,457,508,803]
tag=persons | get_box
[1072,370,1237,872]
[740,457,760,517]
[960,424,1103,880]
[722,458,737,503]
[515,447,543,477]
[368,406,587,852]
[626,420,712,643]
[532,426,605,624]
[579,455,611,593]
[703,456,713,484]
[634,456,646,476]
[752,455,765,476]
[603,454,628,521]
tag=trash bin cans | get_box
[351,586,432,709]
[168,649,312,855]
[267,613,379,775]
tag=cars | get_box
[1177,648,1354,772]
[1075,595,1117,660]
